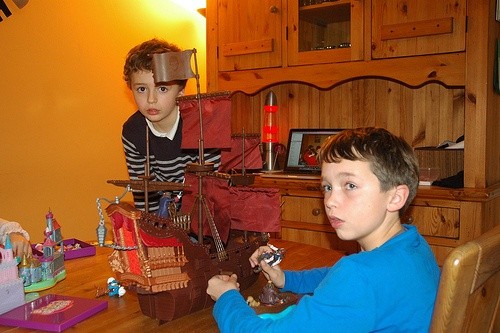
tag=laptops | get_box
[259,127,348,179]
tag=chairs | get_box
[429,225,500,333]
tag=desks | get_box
[0,233,347,333]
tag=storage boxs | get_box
[29,238,96,262]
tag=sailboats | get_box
[94,47,272,322]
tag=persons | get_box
[0,218,32,259]
[122,40,222,213]
[206,127,441,333]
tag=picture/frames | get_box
[283,128,347,174]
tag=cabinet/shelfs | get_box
[207,0,500,270]
